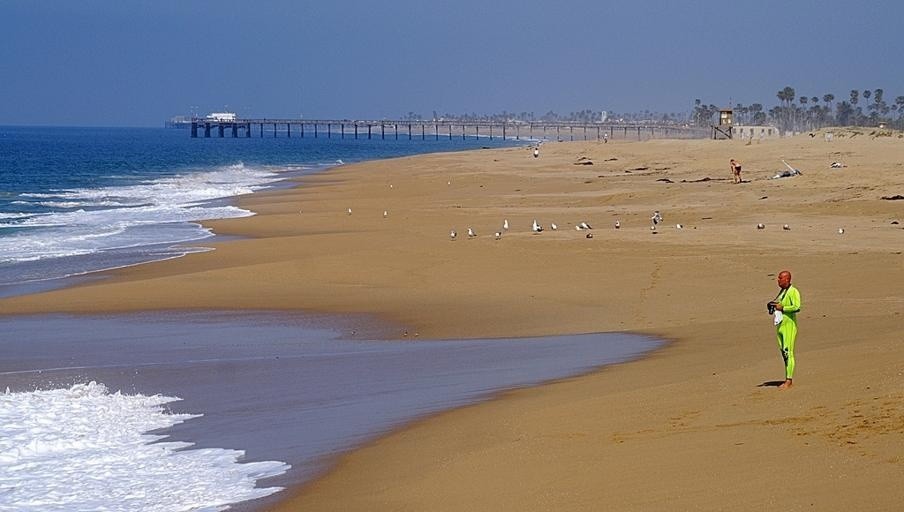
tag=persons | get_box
[730,158,742,184]
[773,271,800,391]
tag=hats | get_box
[773,310,783,326]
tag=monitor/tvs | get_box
[767,301,778,314]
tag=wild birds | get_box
[504,219,509,232]
[587,233,592,238]
[450,230,457,240]
[552,222,557,230]
[575,222,592,231]
[650,211,664,230]
[533,219,544,232]
[495,232,501,240]
[467,227,477,239]
